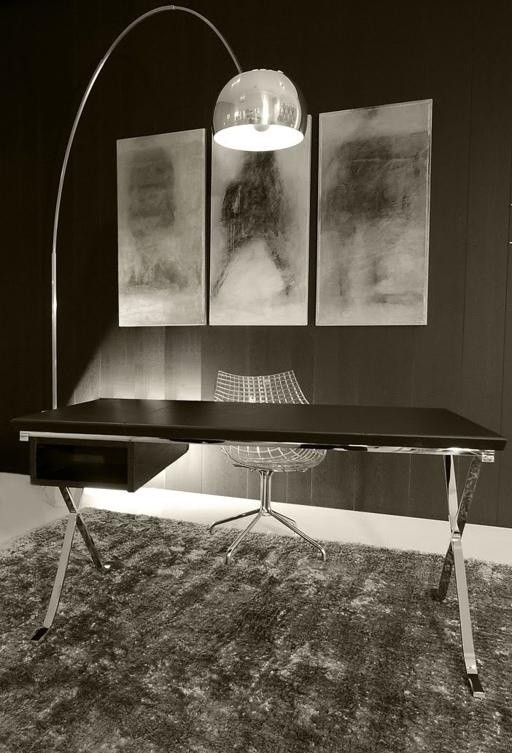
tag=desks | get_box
[19,399,507,709]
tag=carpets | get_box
[1,507,512,753]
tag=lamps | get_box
[44,0,306,413]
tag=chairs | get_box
[211,368,333,568]
